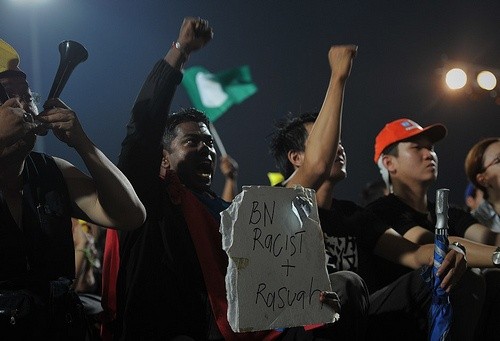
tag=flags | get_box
[181,67,260,123]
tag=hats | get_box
[0,38,26,78]
[374,119,446,165]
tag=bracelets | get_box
[171,40,190,62]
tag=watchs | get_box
[493,246,500,267]
[451,241,466,256]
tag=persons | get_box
[67,219,120,341]
[365,117,500,341]
[217,156,238,208]
[266,45,486,341]
[103,17,342,341]
[0,39,146,341]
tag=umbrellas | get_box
[428,188,452,341]
